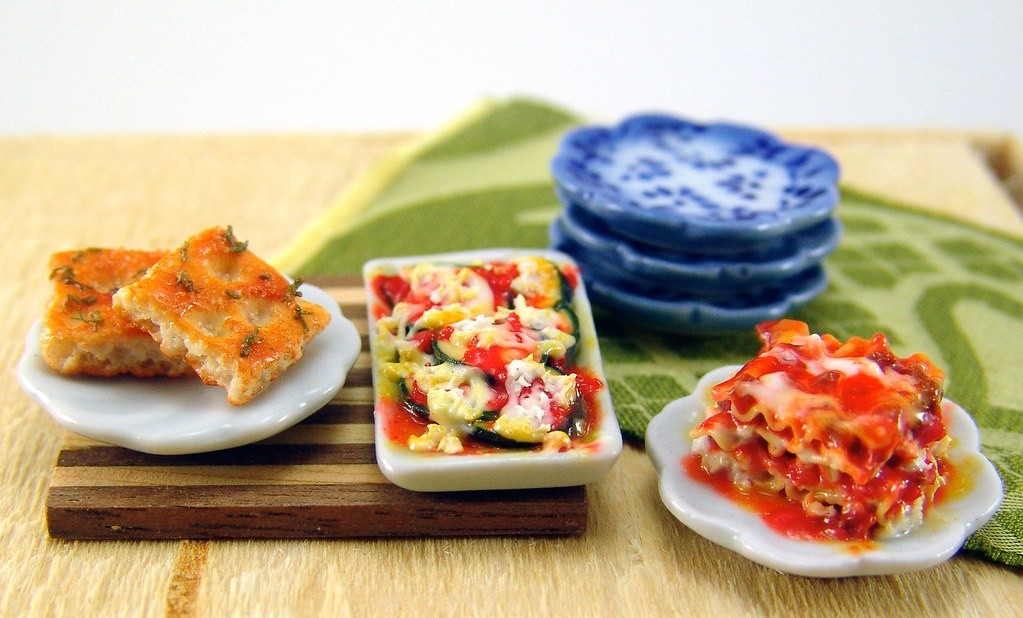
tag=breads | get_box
[112,224,331,404]
[41,248,204,380]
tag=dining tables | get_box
[0,131,1022,616]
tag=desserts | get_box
[688,315,949,541]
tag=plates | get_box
[363,250,624,494]
[18,276,362,458]
[547,117,840,329]
[644,361,1008,583]
